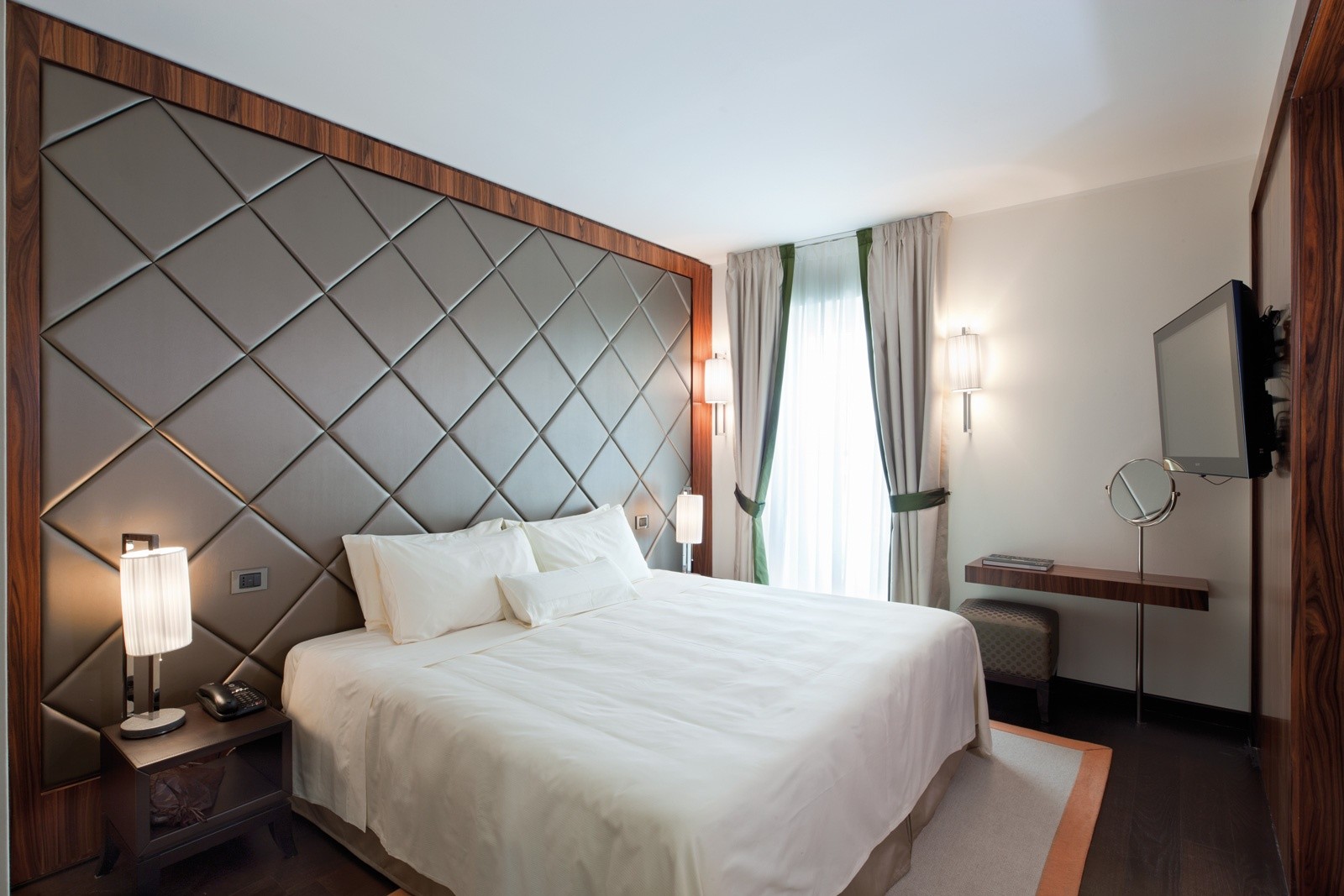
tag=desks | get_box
[965,555,1215,726]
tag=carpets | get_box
[878,715,1115,895]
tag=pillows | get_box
[500,502,613,527]
[342,515,505,631]
[371,521,539,644]
[523,504,655,588]
[495,552,641,629]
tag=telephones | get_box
[192,679,269,722]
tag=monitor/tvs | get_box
[1152,274,1289,481]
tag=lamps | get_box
[947,325,982,434]
[674,489,703,572]
[116,527,197,737]
[703,351,736,435]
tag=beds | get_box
[275,563,997,894]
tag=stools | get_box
[957,598,1064,726]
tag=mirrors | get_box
[1104,456,1180,580]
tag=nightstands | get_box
[92,691,299,887]
